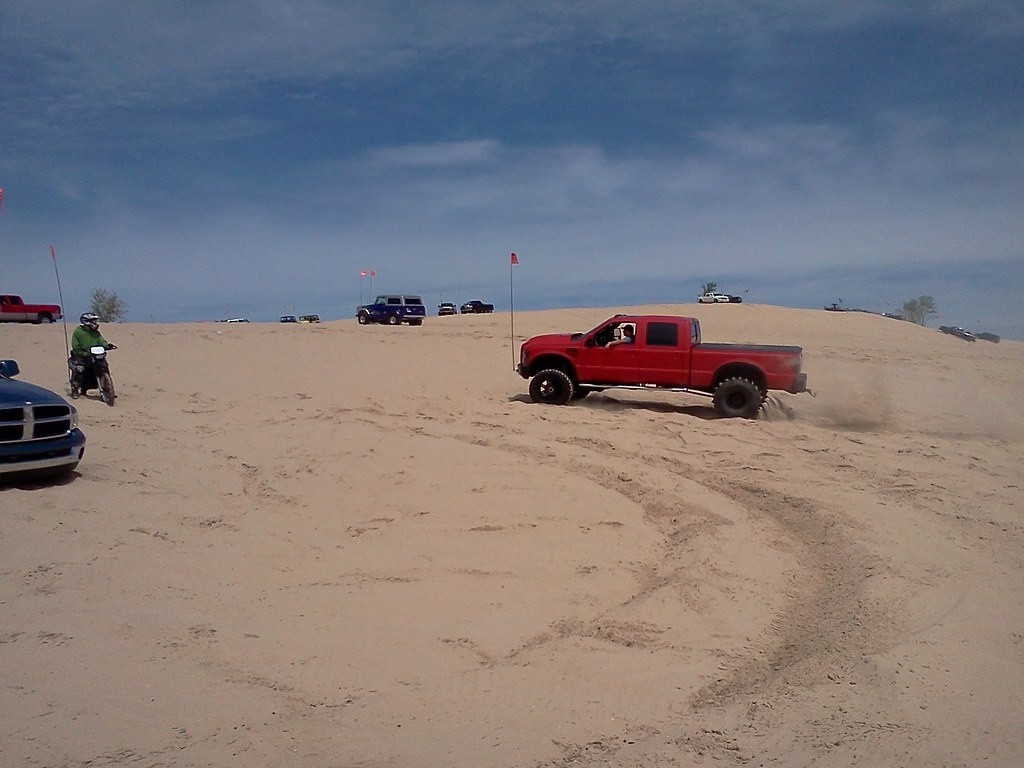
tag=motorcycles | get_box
[66,345,117,407]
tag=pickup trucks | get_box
[939,325,1001,345]
[460,300,494,314]
[0,295,63,323]
[697,292,742,303]
[515,312,816,418]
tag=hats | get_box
[621,325,634,330]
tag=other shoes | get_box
[71,388,78,399]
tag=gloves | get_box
[106,343,114,350]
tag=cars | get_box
[0,359,87,483]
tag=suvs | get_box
[355,294,426,325]
[437,302,458,316]
[280,314,320,324]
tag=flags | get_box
[511,253,518,263]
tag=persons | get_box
[70,313,117,399]
[605,325,635,348]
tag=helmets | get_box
[80,313,101,330]
